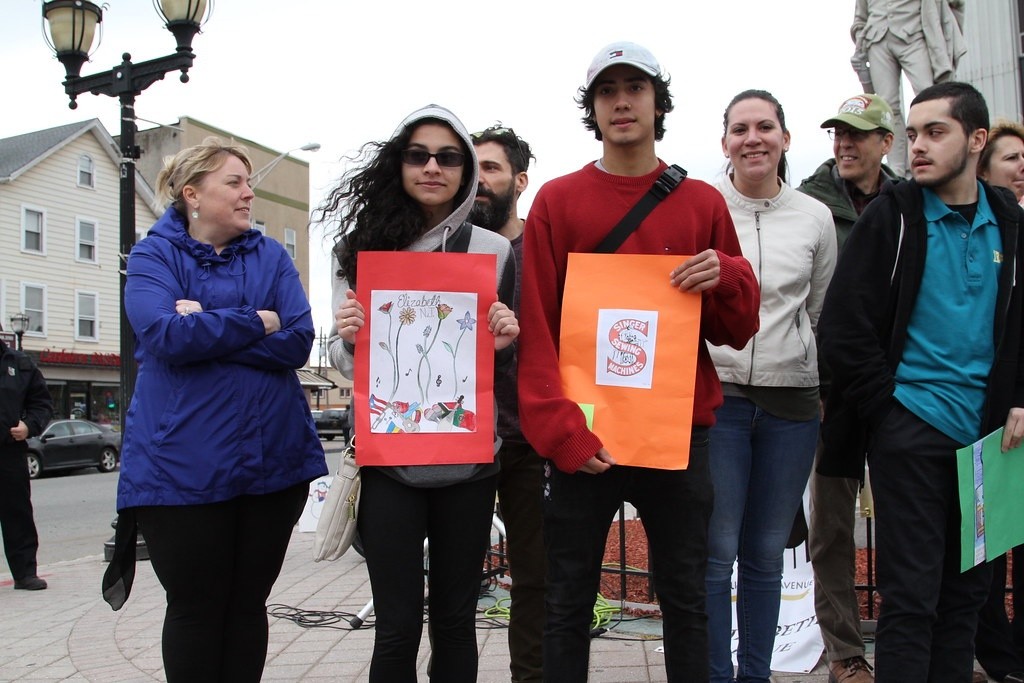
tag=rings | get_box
[186,307,189,314]
[343,318,347,327]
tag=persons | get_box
[817,82,1024,683]
[705,91,836,683]
[794,96,906,683]
[116,141,329,683]
[0,338,47,589]
[850,1,967,177]
[469,129,539,683]
[520,40,761,682]
[308,105,519,683]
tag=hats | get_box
[821,94,895,134]
[585,41,660,91]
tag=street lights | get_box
[41,0,208,565]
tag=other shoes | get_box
[14,578,48,590]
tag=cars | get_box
[316,407,348,440]
[26,419,122,480]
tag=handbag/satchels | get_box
[312,447,361,562]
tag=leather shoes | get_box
[829,656,875,682]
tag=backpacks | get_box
[349,398,502,485]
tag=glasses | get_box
[828,128,887,141]
[401,149,465,167]
[470,128,527,165]
